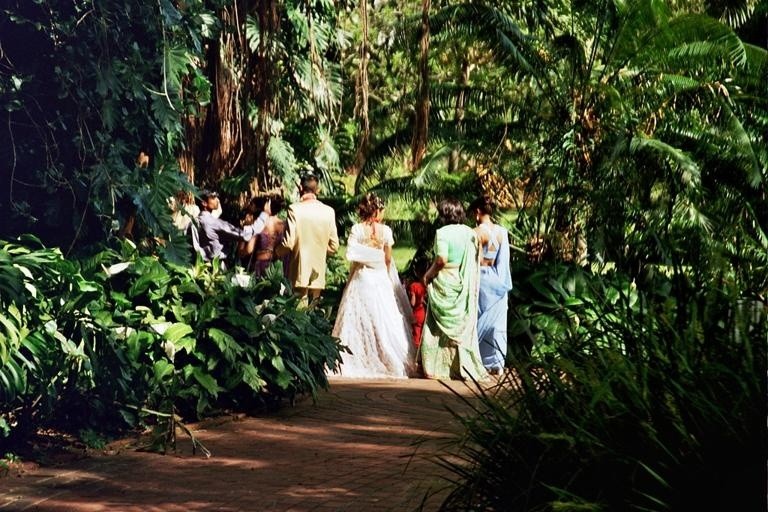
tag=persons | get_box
[416,195,490,383]
[319,190,419,380]
[406,256,429,349]
[467,194,514,376]
[155,188,287,285]
[270,175,340,309]
